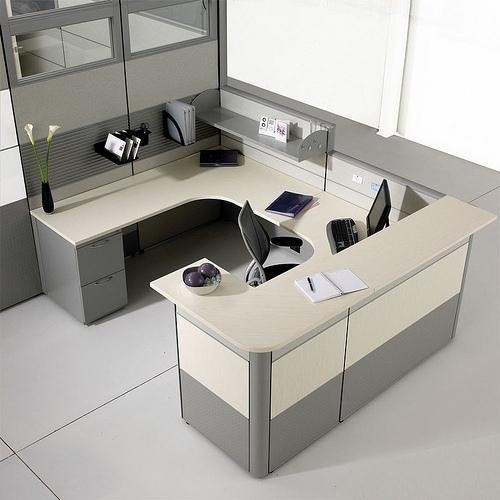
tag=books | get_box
[294,269,368,303]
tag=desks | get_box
[30,144,500,479]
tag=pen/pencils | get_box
[307,277,313,291]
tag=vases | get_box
[42,182,54,215]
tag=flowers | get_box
[24,123,63,183]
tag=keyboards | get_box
[326,218,358,255]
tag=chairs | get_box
[237,200,303,288]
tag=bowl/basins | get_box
[182,266,223,296]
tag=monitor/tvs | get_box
[365,179,391,237]
